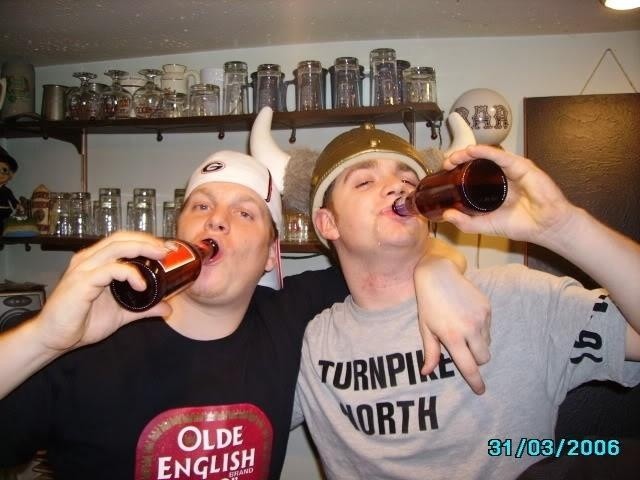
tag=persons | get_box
[288,128,640,480]
[0,150,490,480]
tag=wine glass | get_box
[71,70,166,120]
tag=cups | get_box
[190,48,436,118]
[42,84,68,122]
[164,93,189,118]
[47,188,187,239]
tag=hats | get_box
[309,127,431,250]
[183,151,284,291]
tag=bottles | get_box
[108,239,220,312]
[160,65,201,115]
[392,159,509,221]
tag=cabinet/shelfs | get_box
[0,100,444,256]
